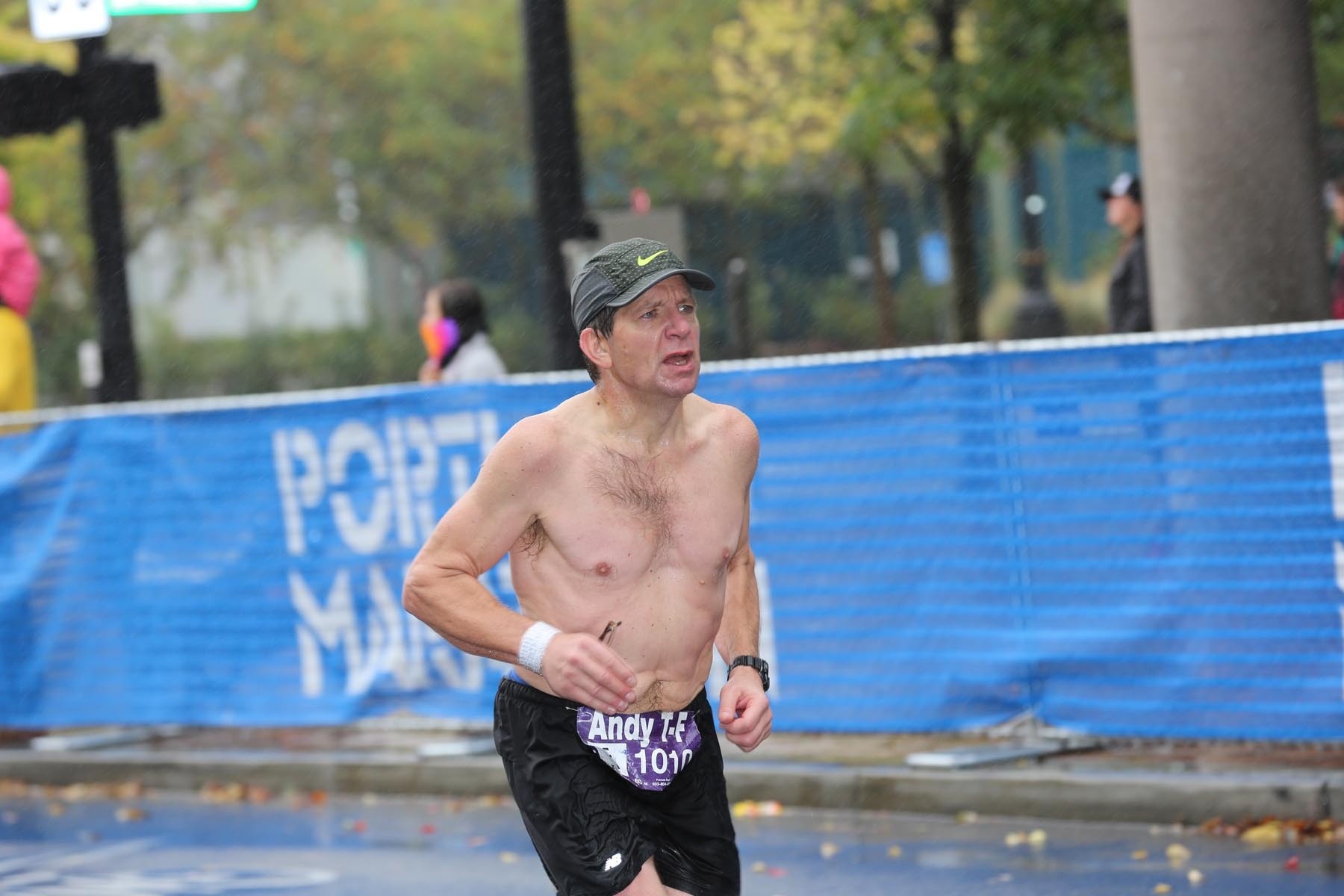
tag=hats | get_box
[571,237,716,333]
[1096,172,1140,200]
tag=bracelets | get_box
[517,621,563,676]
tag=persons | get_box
[0,169,38,435]
[1000,251,1070,339]
[403,237,772,896]
[416,279,504,385]
[1094,173,1151,333]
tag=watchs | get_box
[727,655,770,692]
